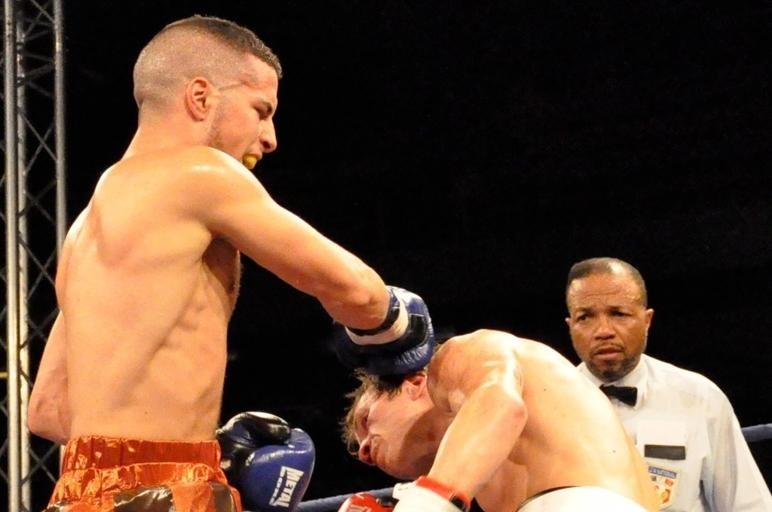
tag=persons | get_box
[565,257,772,512]
[28,16,435,512]
[342,327,659,512]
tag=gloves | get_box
[337,473,471,512]
[333,283,435,378]
[215,410,316,512]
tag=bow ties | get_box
[597,384,636,407]
